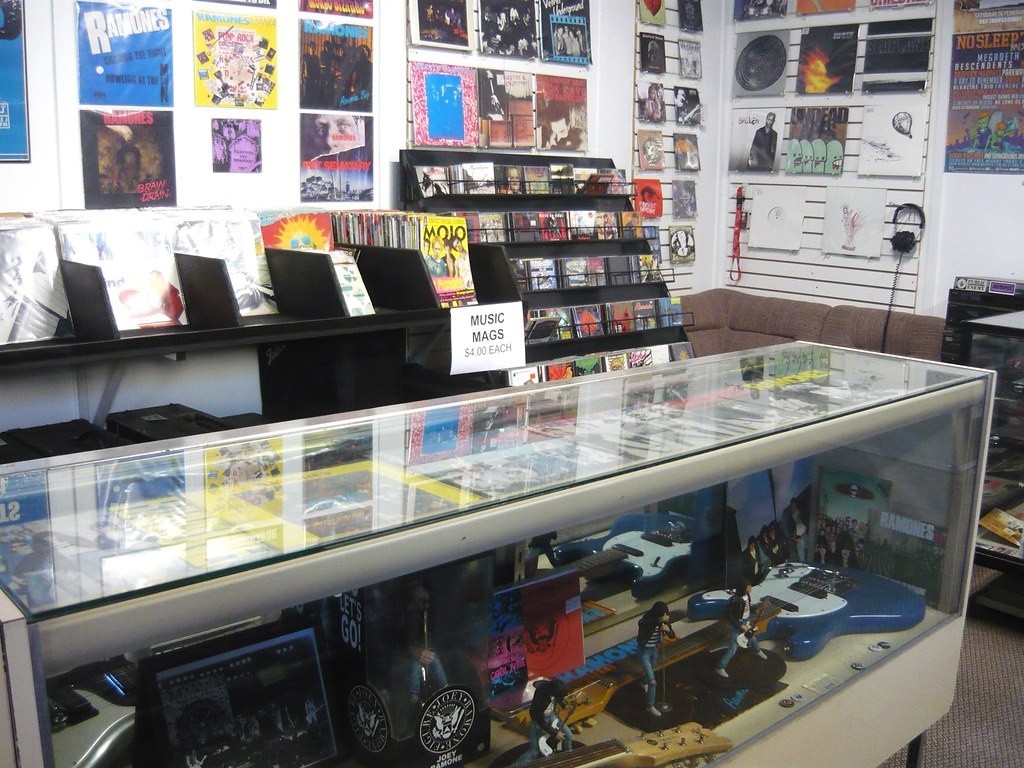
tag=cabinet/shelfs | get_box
[400,150,695,388]
[958,313,1024,629]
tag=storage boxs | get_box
[0,402,275,466]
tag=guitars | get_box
[488,554,922,733]
[507,719,730,768]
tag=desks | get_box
[0,305,452,429]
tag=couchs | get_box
[678,289,945,362]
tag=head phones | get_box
[889,203,926,253]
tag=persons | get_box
[178,691,319,768]
[525,533,588,594]
[815,515,871,568]
[529,677,573,750]
[544,213,561,239]
[113,137,141,192]
[747,497,808,585]
[302,39,373,111]
[400,582,448,704]
[423,231,474,290]
[543,99,586,150]
[313,114,363,154]
[643,0,789,257]
[425,4,587,58]
[715,580,768,677]
[624,214,640,238]
[635,601,675,716]
[499,167,522,194]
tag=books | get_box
[421,215,477,305]
[0,207,436,347]
